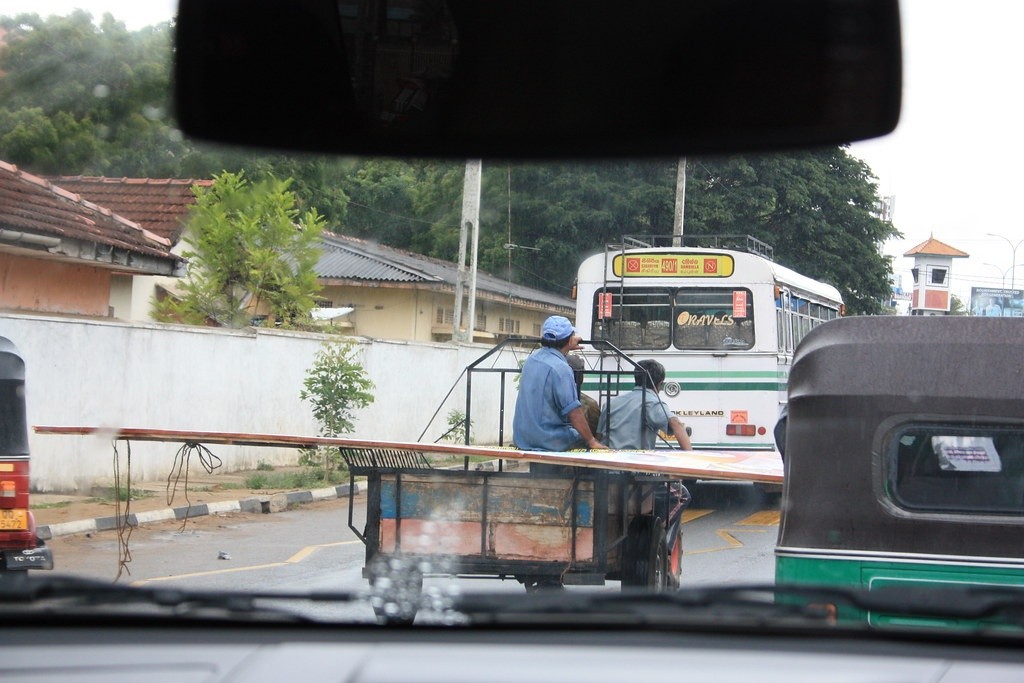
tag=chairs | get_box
[595,321,753,348]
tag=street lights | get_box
[983,233,1024,316]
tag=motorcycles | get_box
[0,336,54,589]
[772,314,1024,639]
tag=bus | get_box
[573,232,846,491]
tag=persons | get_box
[567,354,600,438]
[595,359,692,588]
[513,315,609,453]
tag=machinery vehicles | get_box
[341,341,691,625]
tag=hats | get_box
[565,353,585,371]
[542,316,575,341]
